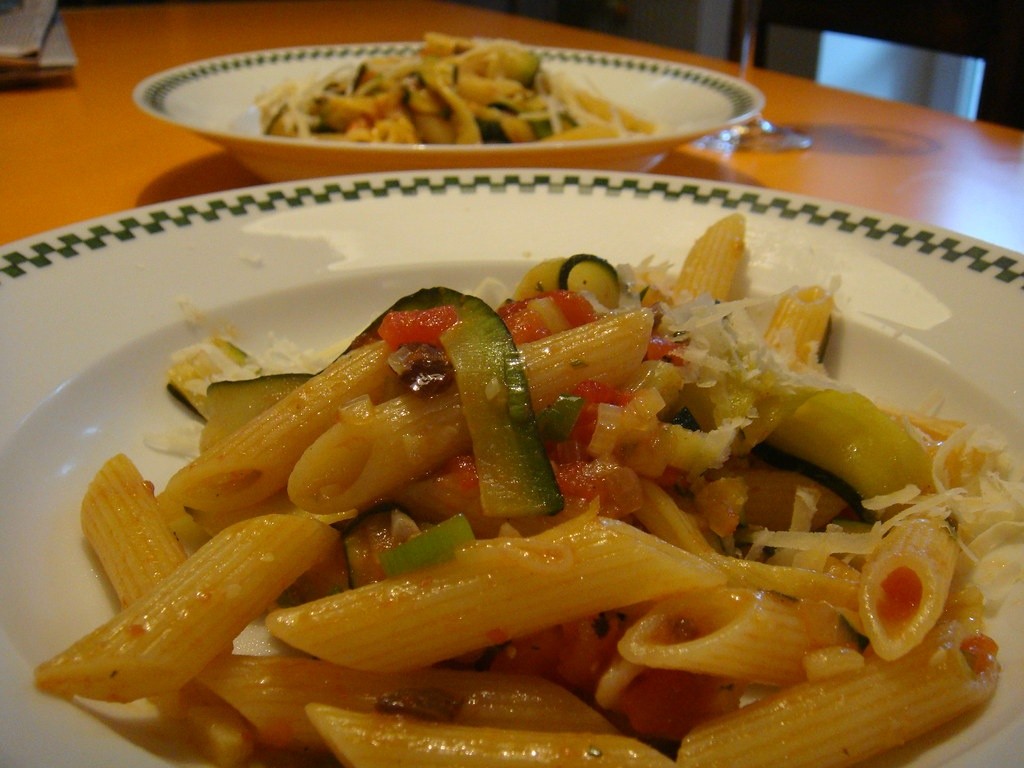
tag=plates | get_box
[130,41,766,182]
[0,168,1024,768]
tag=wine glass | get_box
[691,0,813,153]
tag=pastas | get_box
[262,33,658,146]
[28,214,1002,768]
